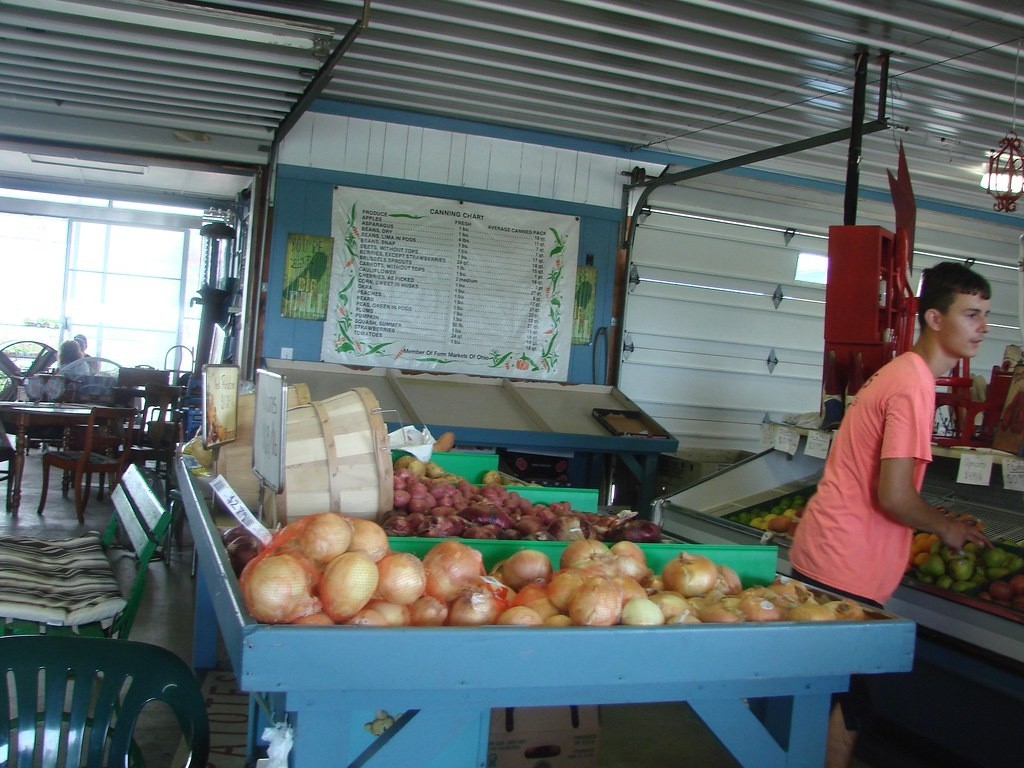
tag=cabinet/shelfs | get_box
[262,356,679,523]
[173,442,919,768]
[651,410,1024,704]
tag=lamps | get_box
[979,130,1023,212]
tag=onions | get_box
[240,469,876,636]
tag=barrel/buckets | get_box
[214,379,313,513]
[262,386,396,529]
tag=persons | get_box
[787,262,993,768]
[56,333,102,379]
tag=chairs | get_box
[0,364,193,527]
[0,632,211,768]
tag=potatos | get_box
[395,431,539,486]
